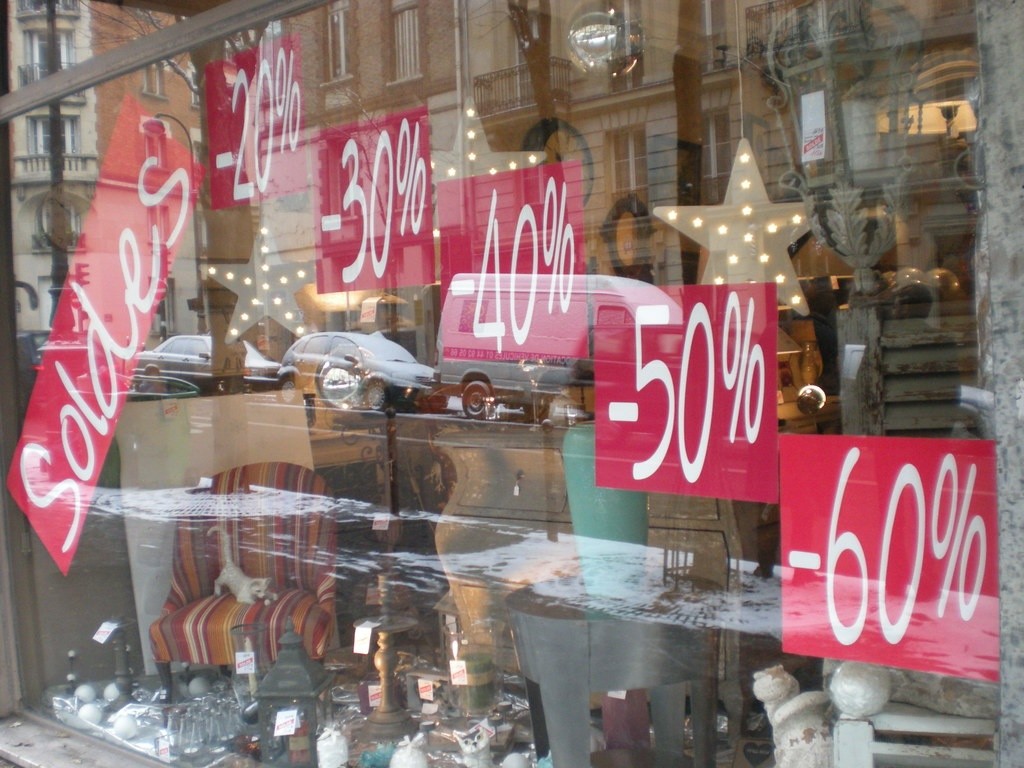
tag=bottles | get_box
[561,422,650,597]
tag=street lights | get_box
[154,110,206,335]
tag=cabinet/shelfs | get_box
[432,429,806,709]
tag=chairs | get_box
[149,461,339,703]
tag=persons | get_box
[880,281,963,439]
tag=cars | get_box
[274,334,447,415]
[16,327,50,378]
[134,333,284,393]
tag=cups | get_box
[167,684,242,768]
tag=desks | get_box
[309,427,390,513]
[503,571,725,768]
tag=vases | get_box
[562,420,648,598]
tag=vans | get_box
[431,273,682,425]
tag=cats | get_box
[206,525,279,606]
